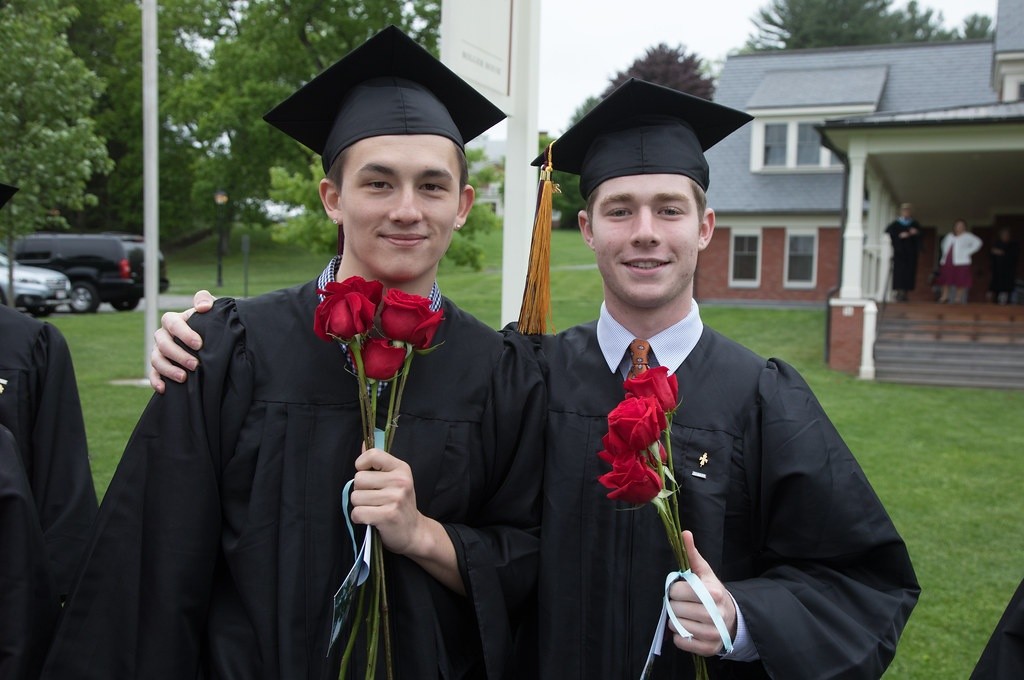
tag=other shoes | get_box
[954,298,962,304]
[940,297,948,302]
[896,294,909,303]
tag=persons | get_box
[882,203,1017,305]
[34,81,552,680]
[0,300,103,680]
[147,126,918,679]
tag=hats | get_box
[262,24,508,256]
[0,183,20,210]
[517,77,756,336]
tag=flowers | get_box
[598,366,717,680]
[313,276,447,680]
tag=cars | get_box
[0,250,73,319]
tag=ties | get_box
[625,338,651,383]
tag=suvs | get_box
[0,232,171,314]
